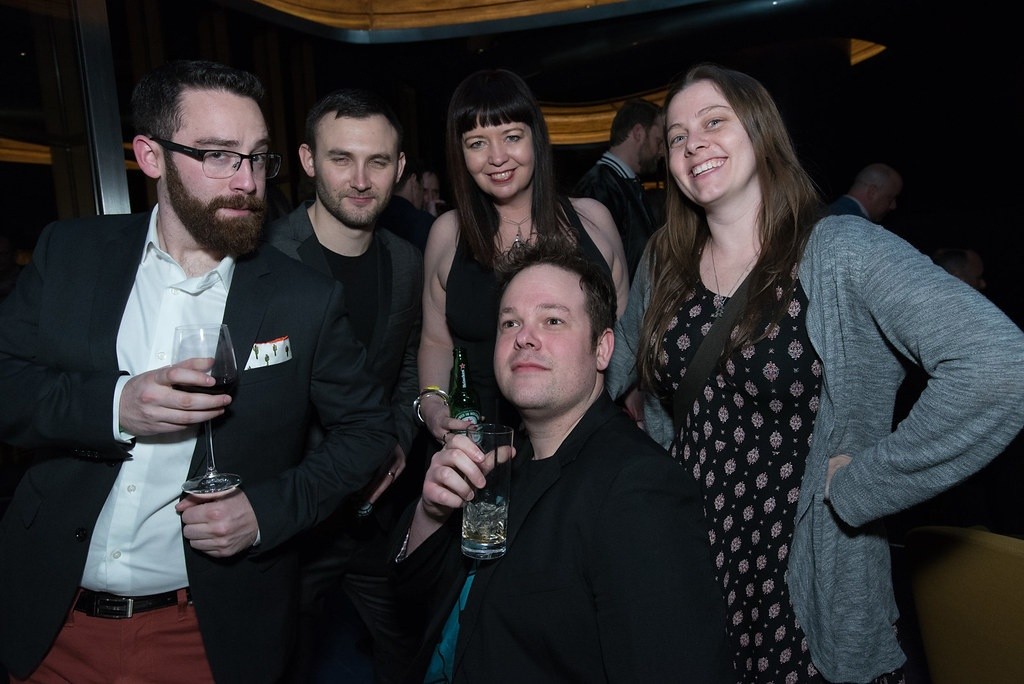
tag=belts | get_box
[72,586,192,619]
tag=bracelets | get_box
[413,385,449,422]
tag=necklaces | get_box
[500,214,531,241]
[710,241,762,307]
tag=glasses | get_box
[149,136,282,181]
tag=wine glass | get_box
[171,322,242,495]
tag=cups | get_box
[461,422,513,560]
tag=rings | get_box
[387,471,395,478]
[443,432,451,443]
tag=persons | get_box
[411,70,629,445]
[602,66,1024,684]
[268,89,423,684]
[938,249,997,306]
[375,238,736,684]
[379,160,446,255]
[828,163,903,224]
[571,97,665,288]
[0,62,400,684]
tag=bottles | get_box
[448,346,484,452]
